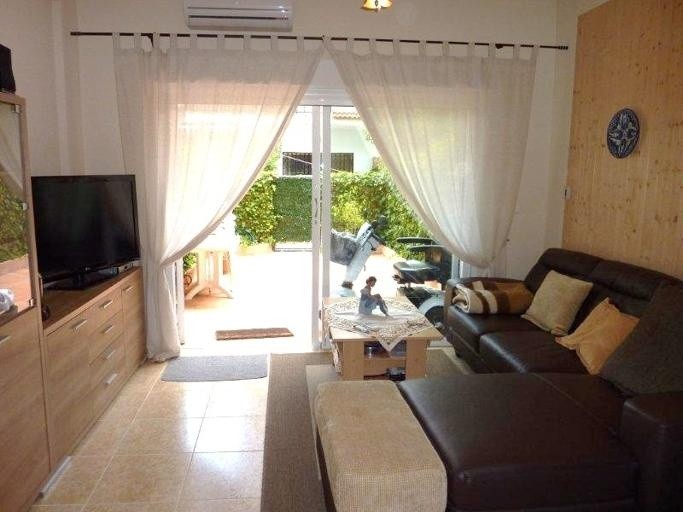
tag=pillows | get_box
[554,297,640,375]
[521,270,594,335]
[597,277,683,397]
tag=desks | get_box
[184,233,241,301]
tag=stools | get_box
[312,380,449,512]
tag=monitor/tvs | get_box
[30,173,142,291]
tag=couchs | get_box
[397,247,683,512]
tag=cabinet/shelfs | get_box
[120,265,148,377]
[43,305,93,473]
[0,305,52,512]
[93,284,127,420]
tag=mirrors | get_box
[0,101,34,327]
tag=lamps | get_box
[362,0,392,13]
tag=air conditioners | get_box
[182,1,295,32]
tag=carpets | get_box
[160,353,268,382]
[260,348,465,512]
[215,327,293,340]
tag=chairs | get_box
[392,234,449,291]
